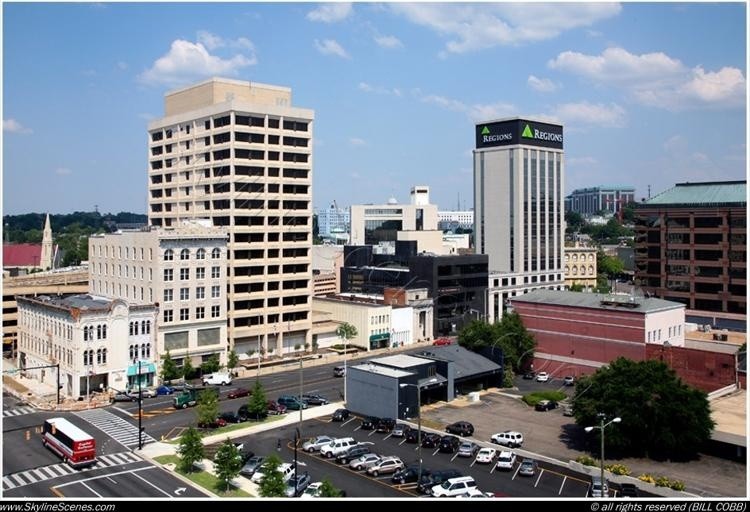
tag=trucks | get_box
[172,387,220,409]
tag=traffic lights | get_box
[41,369,45,383]
[99,383,106,390]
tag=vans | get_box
[334,365,345,377]
[203,373,232,386]
[590,475,608,497]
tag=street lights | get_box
[584,411,621,497]
[337,328,347,402]
[400,379,439,484]
[471,287,495,326]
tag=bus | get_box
[41,418,96,469]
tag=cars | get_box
[563,403,576,416]
[433,337,450,347]
[619,483,637,497]
[535,399,558,411]
[114,381,192,402]
[523,370,576,385]
[227,388,252,399]
